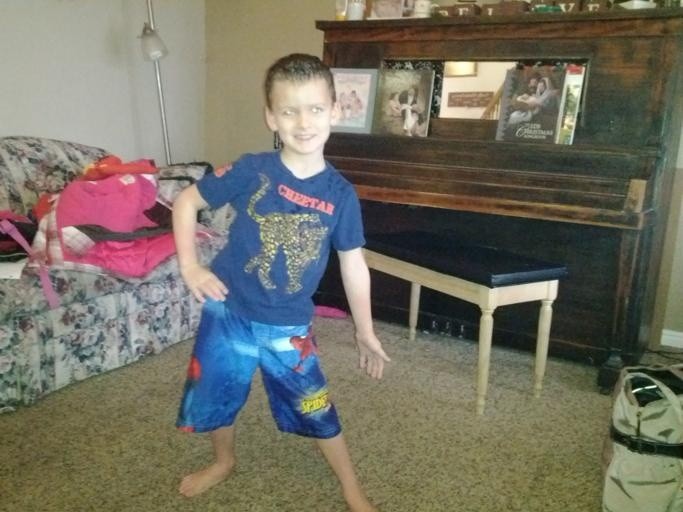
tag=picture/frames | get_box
[329,66,380,134]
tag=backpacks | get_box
[599,361,683,511]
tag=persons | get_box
[399,83,425,136]
[340,87,362,119]
[506,73,559,140]
[384,92,402,117]
[169,51,394,512]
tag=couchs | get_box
[2,132,236,413]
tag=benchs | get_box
[363,230,566,417]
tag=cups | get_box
[334,0,440,21]
